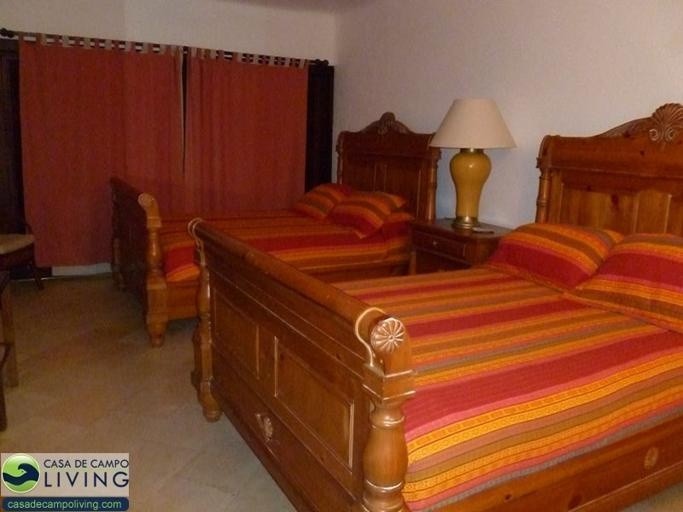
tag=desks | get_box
[0,271,19,431]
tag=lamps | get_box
[428,97,519,230]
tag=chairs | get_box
[0,208,45,292]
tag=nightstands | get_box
[404,217,512,274]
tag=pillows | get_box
[289,182,355,218]
[572,234,682,334]
[326,193,409,238]
[480,222,622,293]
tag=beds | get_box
[188,104,683,512]
[108,111,441,347]
